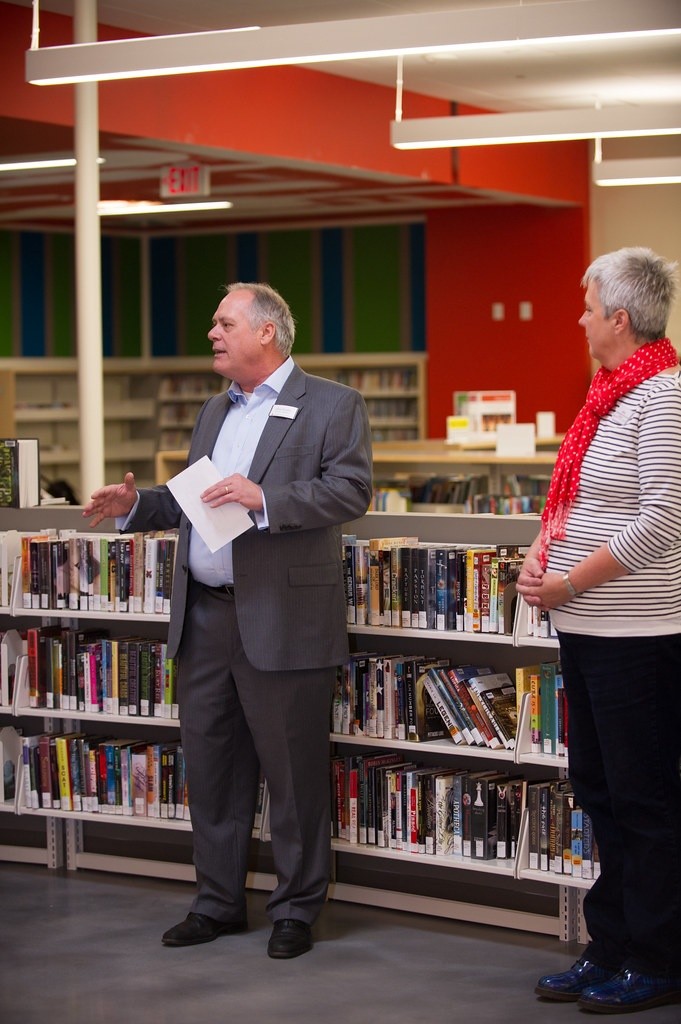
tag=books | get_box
[153,365,550,517]
[0,527,601,880]
[0,436,41,509]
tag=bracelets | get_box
[564,572,578,598]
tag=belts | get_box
[192,580,236,598]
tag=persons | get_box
[515,245,681,1011]
[45,477,80,505]
[80,281,375,959]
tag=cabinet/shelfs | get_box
[0,357,602,944]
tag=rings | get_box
[226,486,228,494]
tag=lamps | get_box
[28,0,681,187]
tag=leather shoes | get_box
[534,952,615,1001]
[576,956,681,1013]
[160,911,248,945]
[267,919,313,959]
